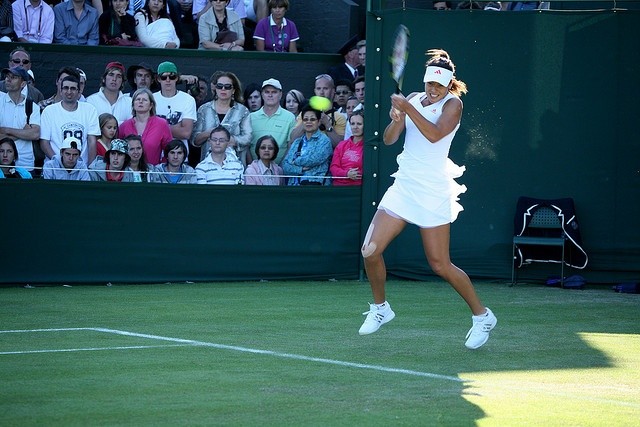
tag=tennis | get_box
[309,95,332,112]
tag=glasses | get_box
[259,146,274,150]
[215,83,234,90]
[62,86,78,92]
[211,137,229,143]
[333,90,351,95]
[315,74,332,79]
[303,118,318,121]
[160,76,177,80]
[9,59,30,64]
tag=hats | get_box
[76,67,86,81]
[105,62,125,74]
[337,35,360,57]
[27,70,34,79]
[262,78,282,90]
[157,61,178,76]
[3,66,28,81]
[105,138,131,170]
[60,137,82,152]
[423,65,453,87]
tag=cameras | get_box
[186,80,199,97]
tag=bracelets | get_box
[232,40,237,46]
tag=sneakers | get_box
[465,307,497,349]
[359,300,395,335]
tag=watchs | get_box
[326,125,334,133]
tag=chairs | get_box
[507,196,575,286]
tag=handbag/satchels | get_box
[214,28,237,44]
[300,180,321,185]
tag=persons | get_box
[0,66,42,179]
[198,0,246,53]
[124,134,150,183]
[243,83,263,111]
[293,74,345,148]
[148,139,197,183]
[74,67,88,104]
[346,94,358,115]
[335,81,353,105]
[90,140,136,182]
[180,70,207,104]
[89,0,104,18]
[245,0,268,29]
[247,77,297,167]
[85,61,134,125]
[137,0,179,48]
[285,88,303,112]
[0,140,32,179]
[192,0,247,28]
[98,0,136,50]
[41,77,102,165]
[355,39,367,70]
[211,70,226,98]
[57,0,101,45]
[244,133,285,187]
[38,65,79,113]
[42,136,90,182]
[94,112,118,157]
[114,87,175,163]
[281,102,333,186]
[355,48,499,349]
[195,124,244,185]
[149,60,197,166]
[356,74,365,107]
[12,0,55,48]
[0,43,42,108]
[330,109,364,187]
[191,71,253,162]
[336,38,360,95]
